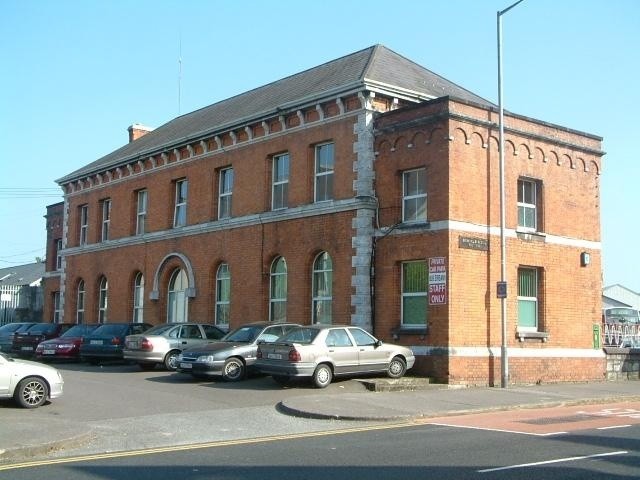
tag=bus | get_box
[603,307,640,346]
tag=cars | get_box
[254,324,417,383]
[13,321,77,354]
[35,322,104,361]
[1,323,31,352]
[1,354,65,408]
[121,320,230,372]
[177,322,305,384]
[79,321,155,365]
